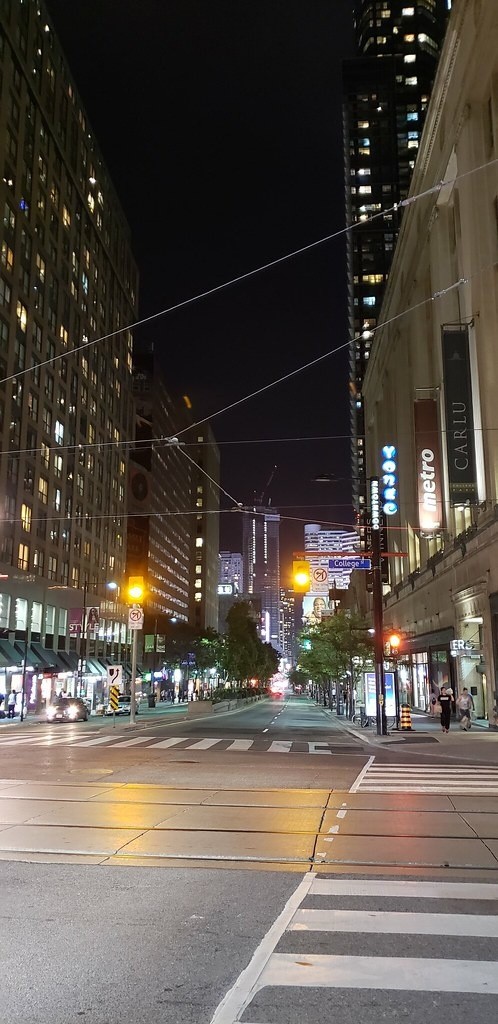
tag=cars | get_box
[106,696,139,716]
[47,697,89,723]
[271,686,283,694]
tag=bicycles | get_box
[322,697,336,707]
[352,712,395,729]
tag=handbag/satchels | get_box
[434,701,442,713]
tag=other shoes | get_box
[442,726,449,733]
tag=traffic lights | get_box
[128,576,144,604]
[390,635,401,657]
[293,562,310,595]
[304,639,311,649]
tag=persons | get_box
[128,690,131,695]
[302,598,326,630]
[348,685,357,712]
[53,688,72,699]
[332,688,337,701]
[437,687,454,734]
[343,690,348,704]
[155,687,217,704]
[456,688,474,731]
[8,690,16,718]
[429,689,437,718]
[87,608,99,632]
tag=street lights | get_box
[210,667,216,700]
[314,473,386,736]
[151,618,178,692]
[80,581,117,696]
[162,662,175,705]
